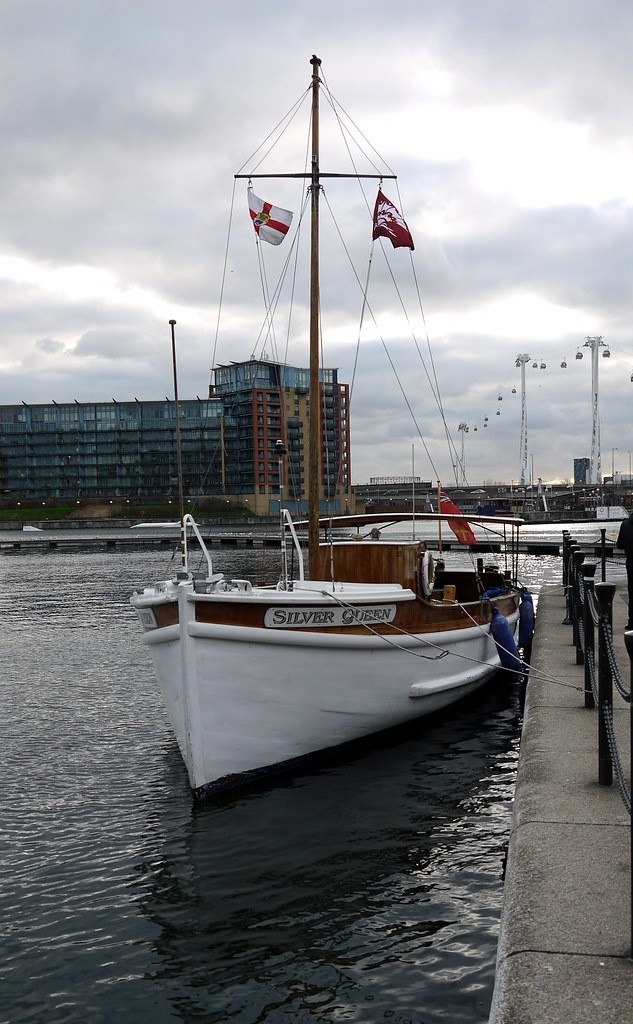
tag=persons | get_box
[616,512,633,631]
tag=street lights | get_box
[573,488,600,508]
[187,499,190,513]
[376,489,380,504]
[515,488,518,512]
[168,498,171,517]
[245,497,248,513]
[278,499,280,512]
[389,497,393,505]
[109,501,113,519]
[522,488,526,502]
[366,488,369,498]
[359,493,366,504]
[405,498,407,506]
[555,491,557,498]
[345,498,348,515]
[17,500,21,521]
[226,498,230,515]
[41,501,46,522]
[76,500,81,519]
[126,500,130,520]
[325,499,329,516]
[297,498,300,516]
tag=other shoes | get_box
[625,625,633,630]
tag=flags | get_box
[371,189,414,251]
[245,189,294,245]
[437,485,479,544]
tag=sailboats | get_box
[128,56,537,810]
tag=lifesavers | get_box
[420,549,436,597]
